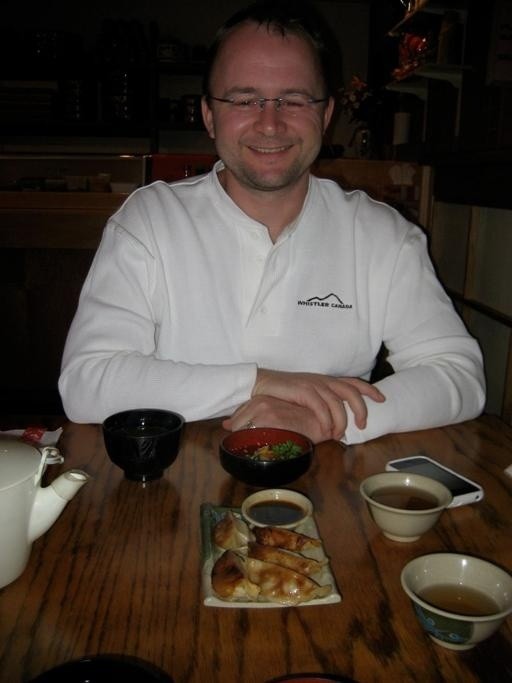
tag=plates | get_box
[199,501,343,610]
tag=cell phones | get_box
[385,455,486,507]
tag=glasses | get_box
[210,92,328,113]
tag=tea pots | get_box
[1,441,91,589]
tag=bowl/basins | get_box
[358,471,453,544]
[239,488,314,530]
[102,408,187,483]
[218,425,317,489]
[399,551,511,652]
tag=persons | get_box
[55,9,487,451]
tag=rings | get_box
[247,421,256,430]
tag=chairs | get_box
[308,158,436,248]
